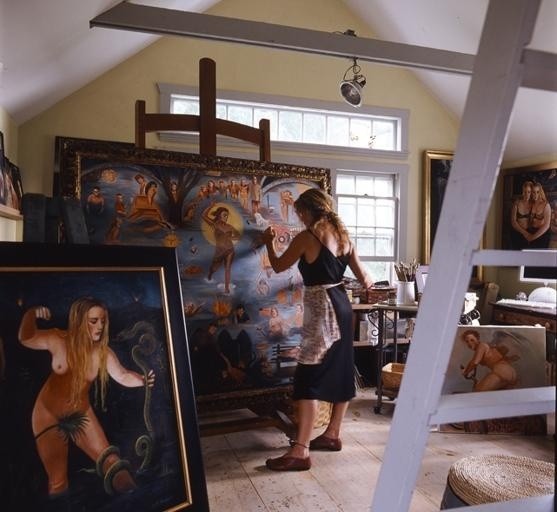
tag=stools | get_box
[440,451,555,512]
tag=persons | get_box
[459,329,519,394]
[16,298,156,502]
[262,184,374,474]
[530,182,553,250]
[510,183,533,248]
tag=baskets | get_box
[380,362,404,397]
[281,396,333,429]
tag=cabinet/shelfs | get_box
[349,302,416,348]
[488,298,556,386]
[374,301,419,409]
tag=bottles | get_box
[387,290,397,306]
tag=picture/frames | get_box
[52,136,337,409]
[493,159,557,250]
[0,241,211,512]
[420,149,485,283]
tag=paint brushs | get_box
[394,259,420,281]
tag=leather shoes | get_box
[265,452,310,471]
[310,433,341,451]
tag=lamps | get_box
[340,28,367,107]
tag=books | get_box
[497,296,556,308]
